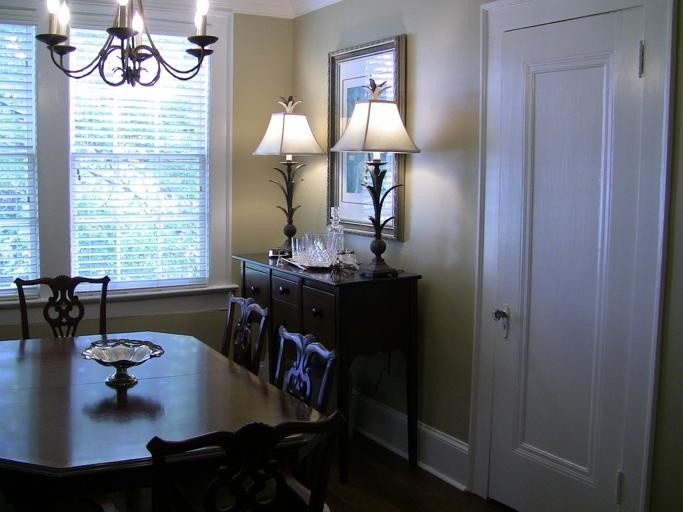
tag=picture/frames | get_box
[328,33,407,241]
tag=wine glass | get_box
[80,338,165,388]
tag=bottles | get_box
[326,207,345,266]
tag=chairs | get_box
[13,275,110,340]
[95,409,347,512]
[273,325,340,410]
[220,290,271,375]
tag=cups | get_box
[291,232,339,270]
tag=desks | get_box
[0,331,327,512]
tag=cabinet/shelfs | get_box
[231,254,422,486]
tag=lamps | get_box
[252,95,326,257]
[35,0,218,87]
[330,79,421,278]
